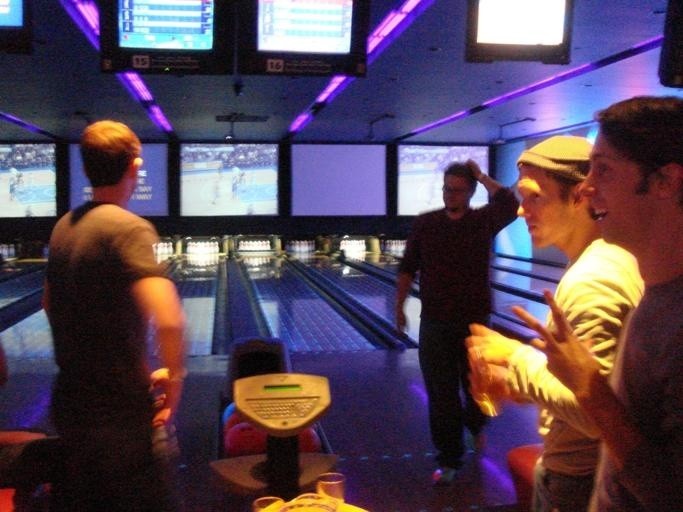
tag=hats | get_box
[516,134,594,183]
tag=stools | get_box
[506,443,544,512]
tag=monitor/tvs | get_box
[0,0,34,56]
[465,0,574,65]
[239,0,369,78]
[658,0,683,90]
[98,0,235,76]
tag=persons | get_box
[40,118,194,511]
[0,143,56,201]
[464,135,644,512]
[433,154,453,178]
[510,95,682,512]
[394,158,522,487]
[181,142,278,205]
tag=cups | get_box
[315,472,346,504]
[466,339,512,417]
[250,496,286,511]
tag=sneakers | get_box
[433,467,454,484]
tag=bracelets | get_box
[477,171,485,182]
